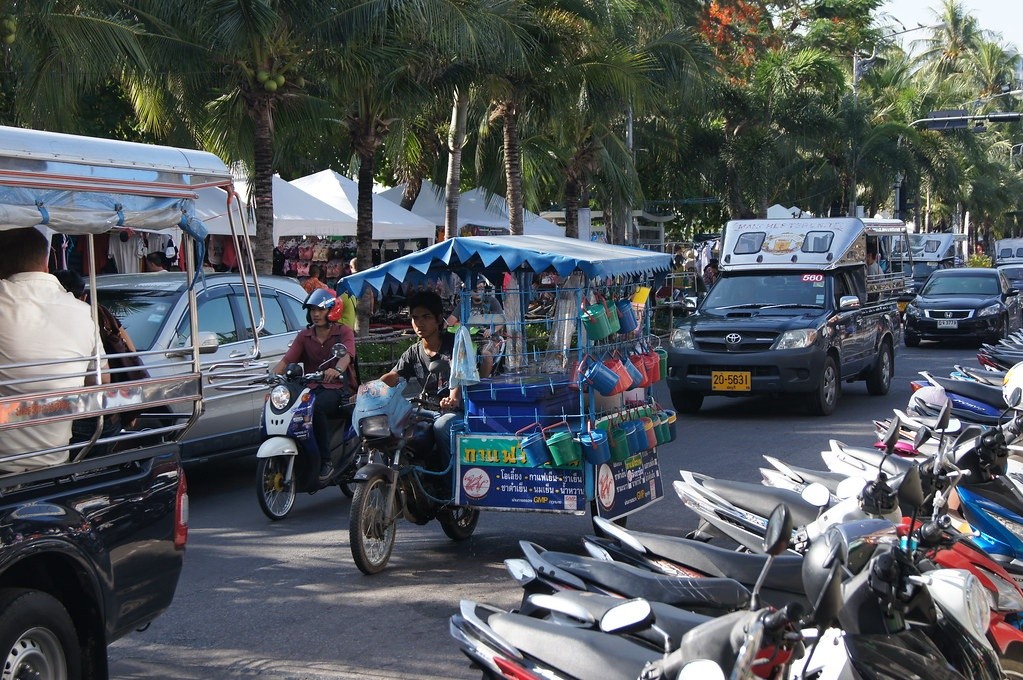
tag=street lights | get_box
[848,21,948,217]
[924,88,1023,233]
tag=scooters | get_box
[447,326,1023,680]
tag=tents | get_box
[161,164,566,251]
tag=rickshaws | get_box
[333,232,676,574]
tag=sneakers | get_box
[317,461,334,481]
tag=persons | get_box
[269,289,354,480]
[868,252,885,279]
[146,255,168,271]
[445,275,506,377]
[302,264,328,295]
[53,269,136,454]
[671,246,707,296]
[352,290,464,500]
[0,227,109,475]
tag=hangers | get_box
[284,236,357,277]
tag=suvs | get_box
[889,232,965,319]
[991,236,1023,293]
[78,266,315,471]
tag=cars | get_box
[901,266,1023,347]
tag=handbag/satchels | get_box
[104,331,155,428]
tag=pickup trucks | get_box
[665,215,914,418]
[0,121,266,680]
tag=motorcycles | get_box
[247,343,373,521]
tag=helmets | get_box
[302,287,344,324]
[905,387,953,419]
[801,516,895,613]
[1001,362,1023,411]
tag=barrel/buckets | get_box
[580,296,612,341]
[515,341,677,467]
[612,291,638,333]
[596,293,621,334]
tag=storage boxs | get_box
[463,372,578,432]
